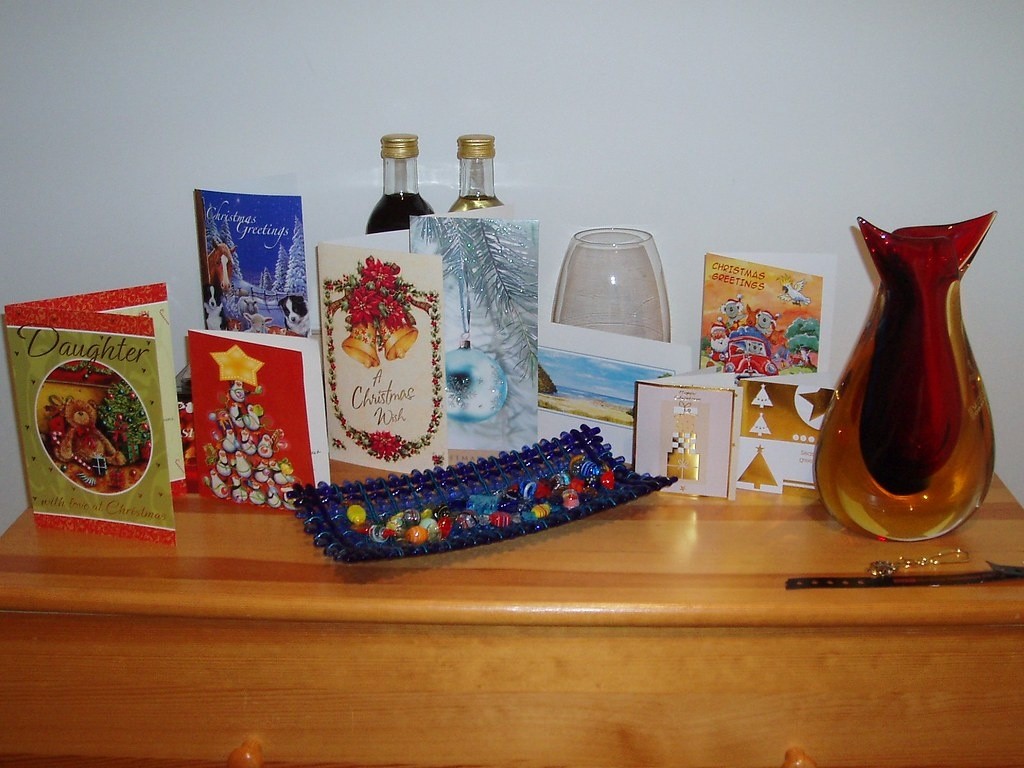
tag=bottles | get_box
[366,133,434,234]
[448,134,504,213]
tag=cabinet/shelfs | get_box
[0,470,1024,768]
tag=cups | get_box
[552,228,671,342]
